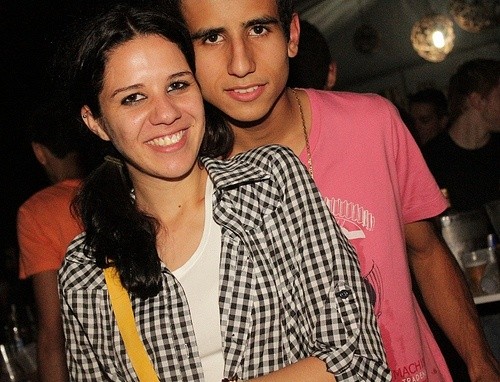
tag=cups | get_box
[462,249,488,296]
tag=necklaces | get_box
[288,88,314,182]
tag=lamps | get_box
[352,0,381,57]
[450,0,500,34]
[409,0,456,63]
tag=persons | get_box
[168,0,500,382]
[288,18,500,249]
[16,94,96,382]
[58,5,392,382]
